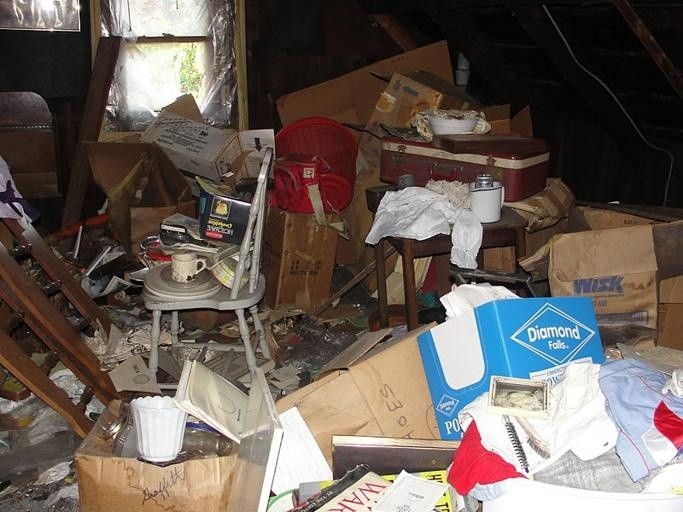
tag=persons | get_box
[507,388,544,410]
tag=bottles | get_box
[183,430,230,456]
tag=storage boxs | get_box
[1,92,63,200]
[518,220,680,351]
[76,399,238,511]
[77,37,566,331]
[573,200,682,232]
[280,321,449,475]
[655,276,682,353]
[413,297,606,444]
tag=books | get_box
[174,361,248,444]
[296,433,462,512]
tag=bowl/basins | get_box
[428,117,477,135]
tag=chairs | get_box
[142,148,274,382]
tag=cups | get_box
[171,253,206,283]
[470,180,505,223]
[130,394,189,463]
